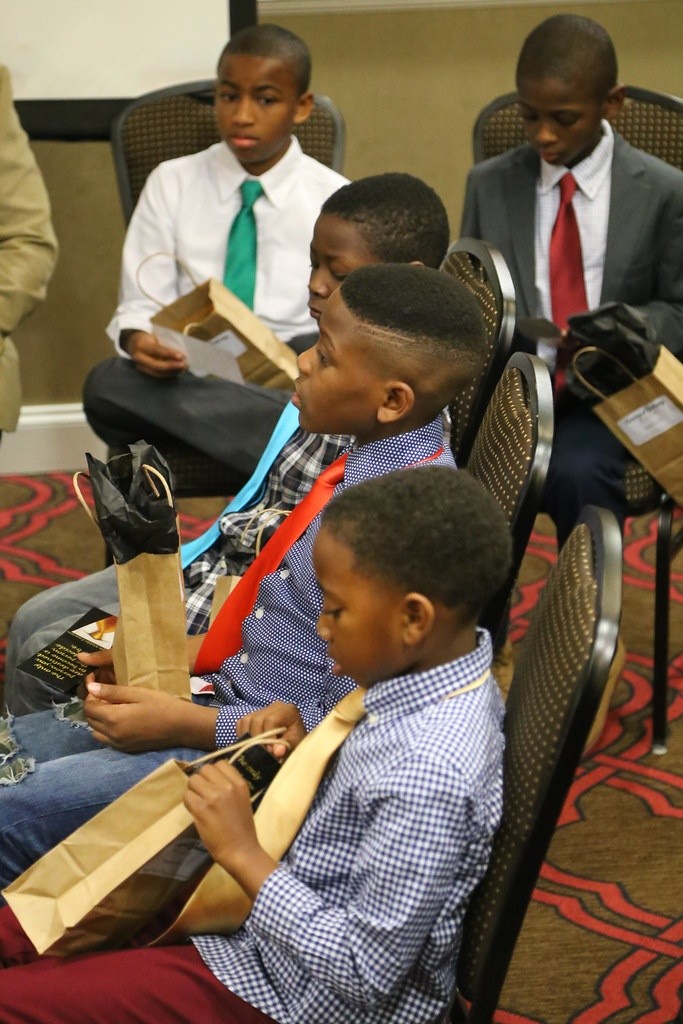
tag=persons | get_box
[0,261,481,896]
[458,13,683,557]
[83,23,365,482]
[0,465,505,1023]
[0,60,58,446]
[6,170,450,714]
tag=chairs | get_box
[450,501,624,1023]
[453,351,555,652]
[470,84,683,171]
[107,78,348,226]
[439,234,518,470]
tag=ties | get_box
[193,451,349,675]
[222,179,264,309]
[144,684,369,946]
[549,172,591,407]
[179,399,302,573]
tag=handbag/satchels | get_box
[135,249,300,397]
[572,342,682,507]
[76,459,193,704]
[0,726,293,959]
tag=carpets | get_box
[0,468,680,1022]
